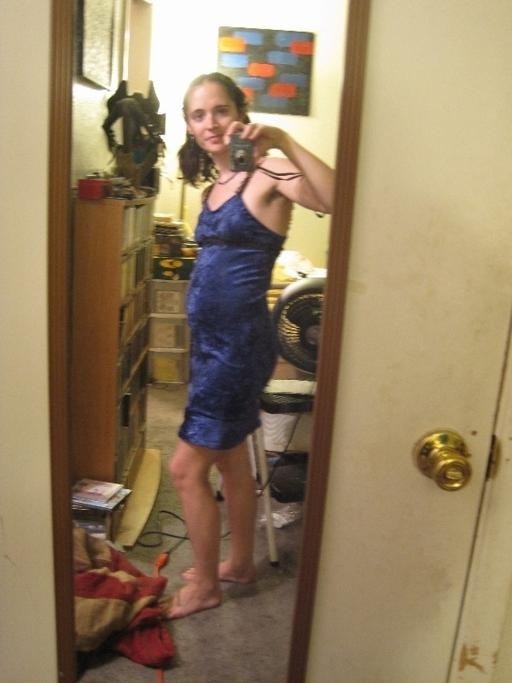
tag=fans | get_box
[273,276,325,379]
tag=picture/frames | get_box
[78,1,115,92]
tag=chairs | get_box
[216,288,314,564]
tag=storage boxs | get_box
[150,278,191,385]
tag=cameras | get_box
[227,133,255,172]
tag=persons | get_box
[166,72,334,620]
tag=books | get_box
[71,477,133,511]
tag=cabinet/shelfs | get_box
[70,195,162,548]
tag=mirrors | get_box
[49,0,368,683]
